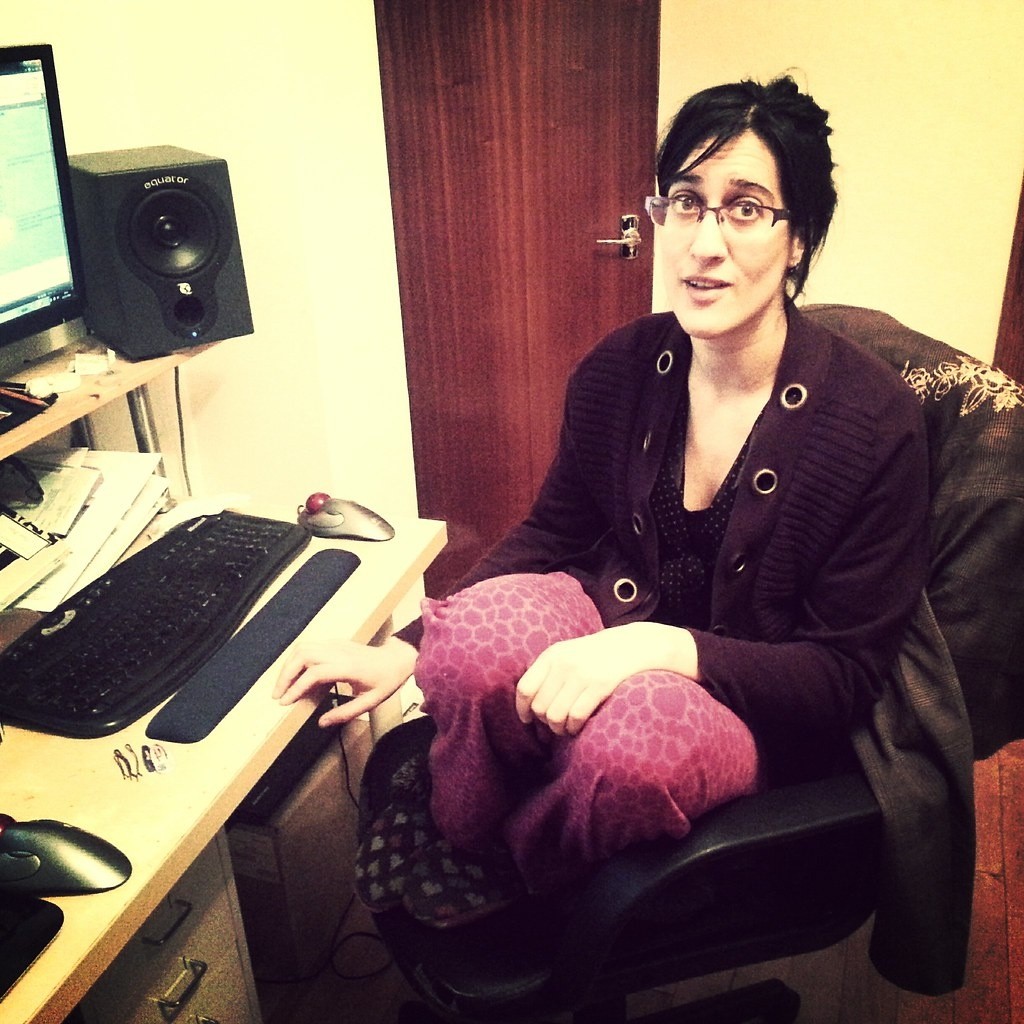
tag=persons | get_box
[273,77,931,926]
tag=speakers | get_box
[70,144,255,363]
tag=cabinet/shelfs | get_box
[79,823,265,1024]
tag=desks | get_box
[0,339,449,1024]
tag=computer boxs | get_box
[223,694,376,985]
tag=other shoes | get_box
[353,754,444,913]
[399,836,527,928]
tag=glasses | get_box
[644,196,791,234]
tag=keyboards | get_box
[0,510,313,740]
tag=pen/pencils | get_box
[0,386,51,408]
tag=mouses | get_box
[0,813,133,892]
[298,493,395,542]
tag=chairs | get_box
[358,304,1024,1024]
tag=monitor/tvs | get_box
[0,43,87,380]
